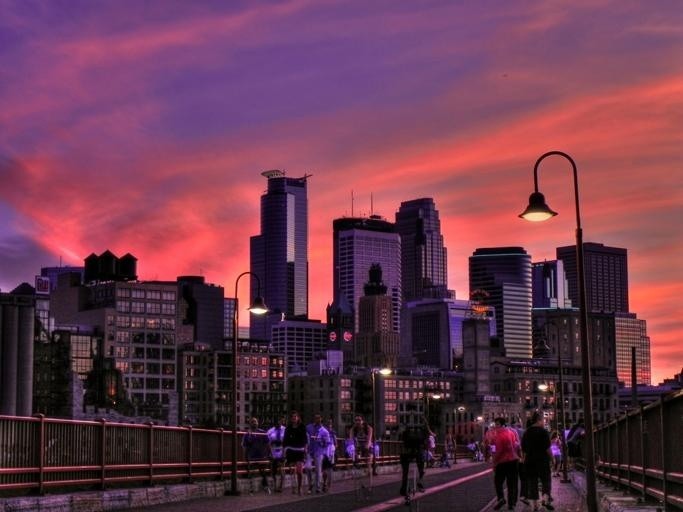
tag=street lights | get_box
[534,319,567,480]
[517,149,596,511]
[230,271,268,493]
[426,394,495,462]
[371,367,391,457]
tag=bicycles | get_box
[352,448,373,506]
[405,458,419,512]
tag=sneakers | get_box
[417,482,425,493]
[400,487,407,496]
[292,486,373,498]
[494,473,560,512]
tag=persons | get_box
[567,423,586,470]
